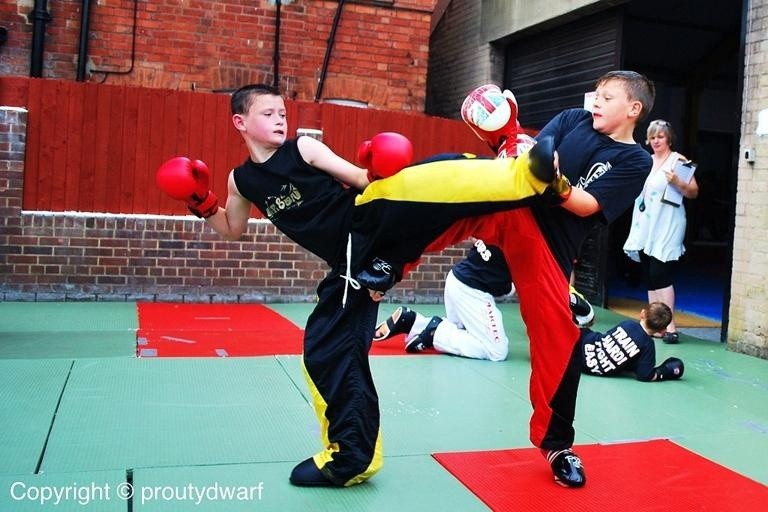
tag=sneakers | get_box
[542,448,585,488]
[372,306,443,354]
[664,332,679,344]
[290,457,325,486]
[357,257,394,289]
[530,135,554,183]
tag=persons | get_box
[575,299,684,381]
[155,82,562,486]
[370,236,595,362]
[623,120,701,344]
[354,68,656,488]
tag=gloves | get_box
[656,356,684,380]
[358,133,414,183]
[570,293,595,326]
[460,84,518,146]
[156,157,219,217]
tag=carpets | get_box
[368,332,444,353]
[431,438,767,511]
[133,304,311,355]
